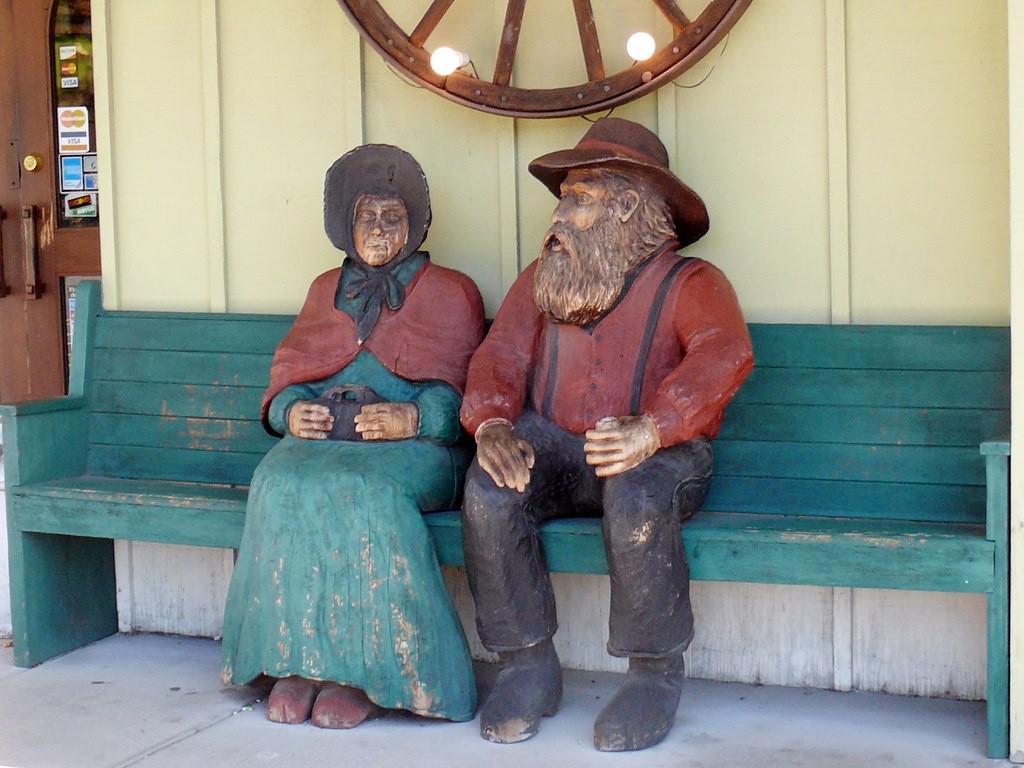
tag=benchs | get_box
[0,279,1011,760]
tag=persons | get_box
[221,143,488,728]
[462,119,753,752]
